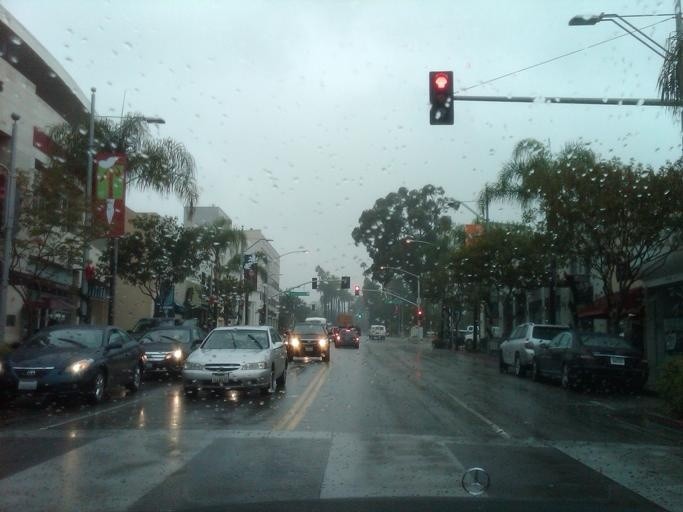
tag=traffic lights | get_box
[417,310,424,321]
[354,285,361,297]
[428,71,455,125]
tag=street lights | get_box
[447,196,484,353]
[240,227,277,326]
[267,244,314,329]
[404,238,455,345]
[378,264,424,340]
[76,83,168,328]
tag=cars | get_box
[125,316,171,343]
[498,320,573,380]
[530,327,651,398]
[284,319,331,363]
[2,321,149,407]
[181,324,289,400]
[335,328,360,350]
[136,325,210,382]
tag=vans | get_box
[368,324,387,341]
